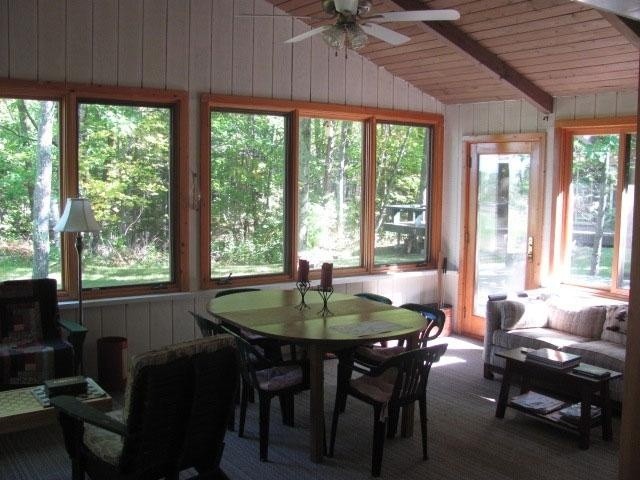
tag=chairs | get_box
[352,292,393,347]
[337,303,445,425]
[50,334,242,479]
[327,344,449,477]
[215,288,260,298]
[216,324,312,462]
[0,278,76,391]
[187,309,267,432]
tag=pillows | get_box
[602,302,628,345]
[499,299,549,329]
[549,298,606,335]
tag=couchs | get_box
[483,285,628,419]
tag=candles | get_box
[297,259,309,281]
[321,262,334,286]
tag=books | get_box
[512,390,601,429]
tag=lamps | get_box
[320,1,372,59]
[52,198,104,325]
[236,0,460,58]
